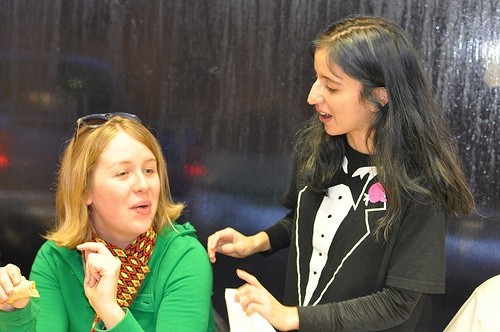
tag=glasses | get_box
[74,112,141,143]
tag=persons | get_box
[207,14,474,332]
[0,113,216,332]
